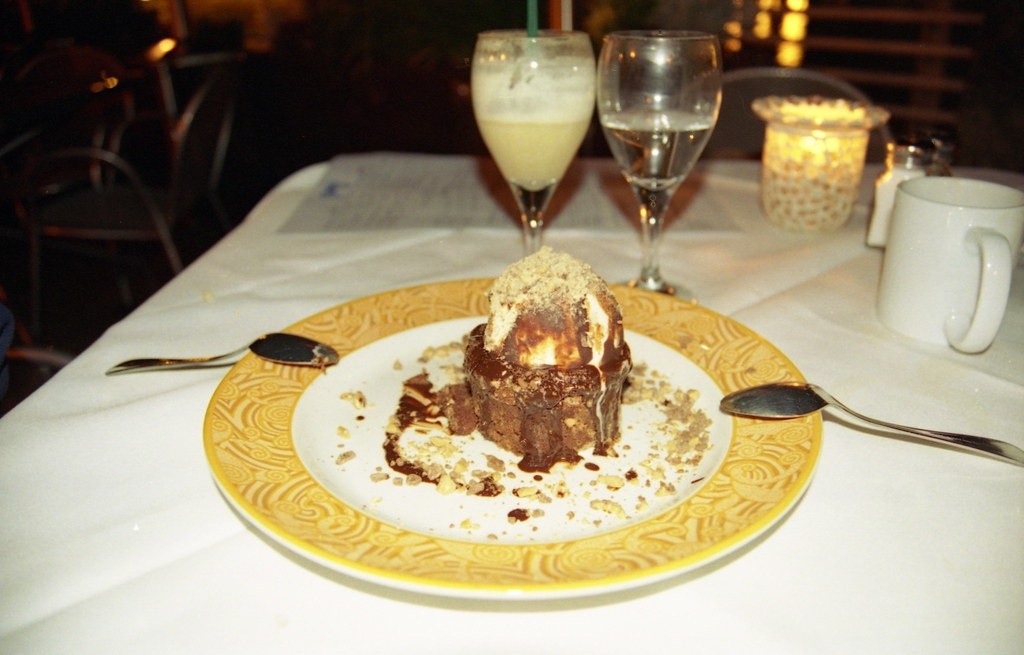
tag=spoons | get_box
[719,381,1024,467]
[106,333,338,378]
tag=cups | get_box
[877,176,1022,358]
[752,95,889,232]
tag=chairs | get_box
[697,68,897,161]
[27,62,238,343]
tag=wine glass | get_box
[472,30,595,274]
[596,31,725,295]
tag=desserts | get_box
[441,245,636,469]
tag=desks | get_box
[0,149,1024,655]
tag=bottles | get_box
[866,135,957,249]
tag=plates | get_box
[204,276,825,600]
